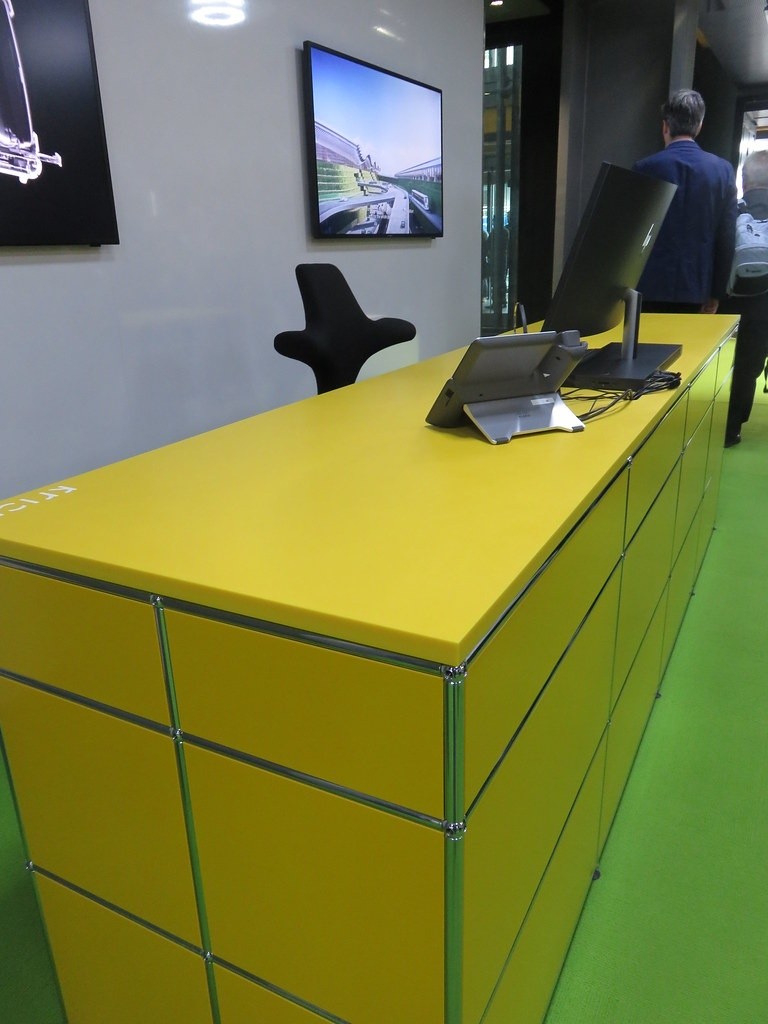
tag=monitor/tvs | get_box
[540,161,683,391]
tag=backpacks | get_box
[725,197,768,298]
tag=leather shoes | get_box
[724,434,741,448]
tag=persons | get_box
[715,151,768,448]
[629,89,738,314]
